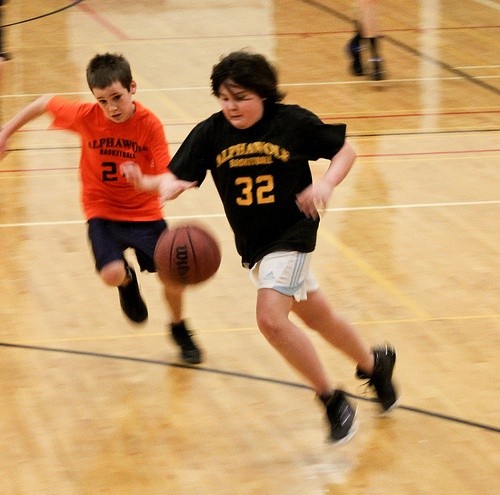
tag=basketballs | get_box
[155,226,221,284]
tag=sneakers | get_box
[318,387,361,445]
[355,344,402,416]
[170,320,201,363]
[117,266,148,323]
[349,33,363,75]
[371,37,382,80]
